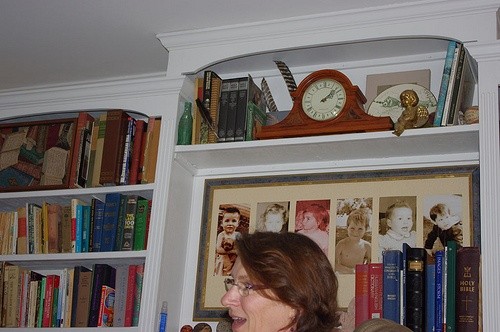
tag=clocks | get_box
[301,78,347,122]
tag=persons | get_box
[392,90,427,136]
[298,204,330,255]
[221,232,339,332]
[259,203,287,234]
[335,211,372,273]
[424,202,461,256]
[215,207,243,276]
[379,200,417,262]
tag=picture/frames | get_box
[191,164,481,323]
[364,70,430,116]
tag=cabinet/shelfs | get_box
[0,0,500,332]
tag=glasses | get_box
[223,278,272,297]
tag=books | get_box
[0,193,151,255]
[355,240,483,332]
[0,261,144,328]
[192,70,265,144]
[69,110,161,189]
[434,42,478,126]
